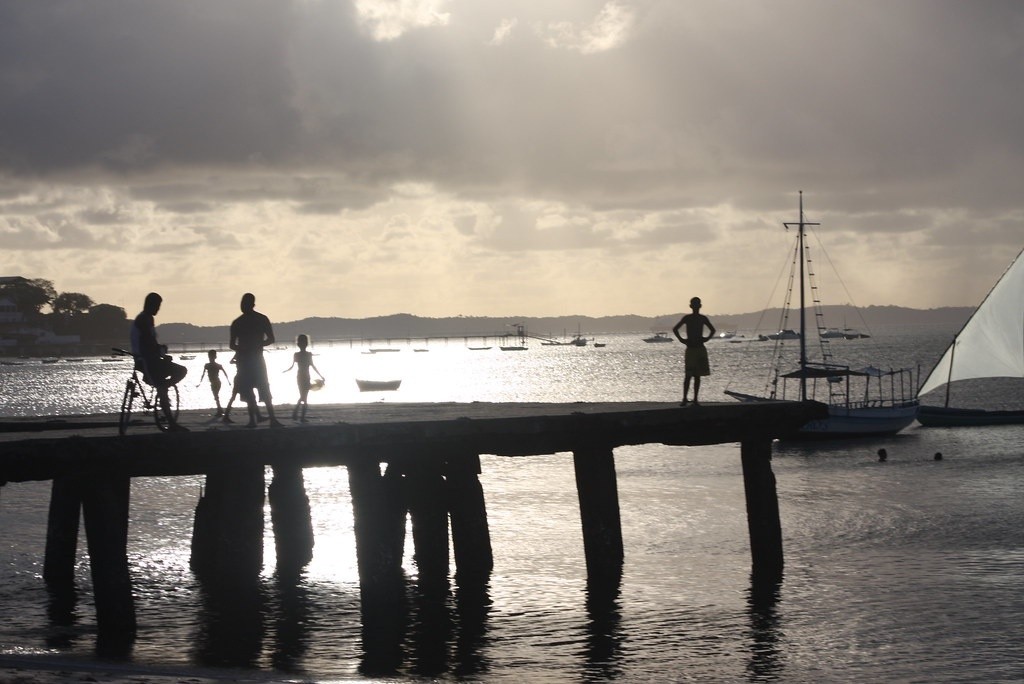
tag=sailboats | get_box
[916,246,1024,425]
[576,322,587,346]
[724,184,924,440]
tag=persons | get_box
[196,350,230,416]
[282,333,325,421]
[672,297,715,405]
[222,351,270,424]
[130,292,190,431]
[229,293,285,429]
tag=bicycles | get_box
[110,345,181,435]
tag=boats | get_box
[66,357,85,362]
[414,349,429,351]
[500,346,529,350]
[641,332,673,343]
[818,327,846,338]
[468,346,493,350]
[100,357,124,363]
[369,348,401,352]
[179,355,197,360]
[767,328,801,340]
[356,378,402,392]
[41,357,59,364]
[594,343,605,347]
[542,341,574,346]
[843,328,870,338]
